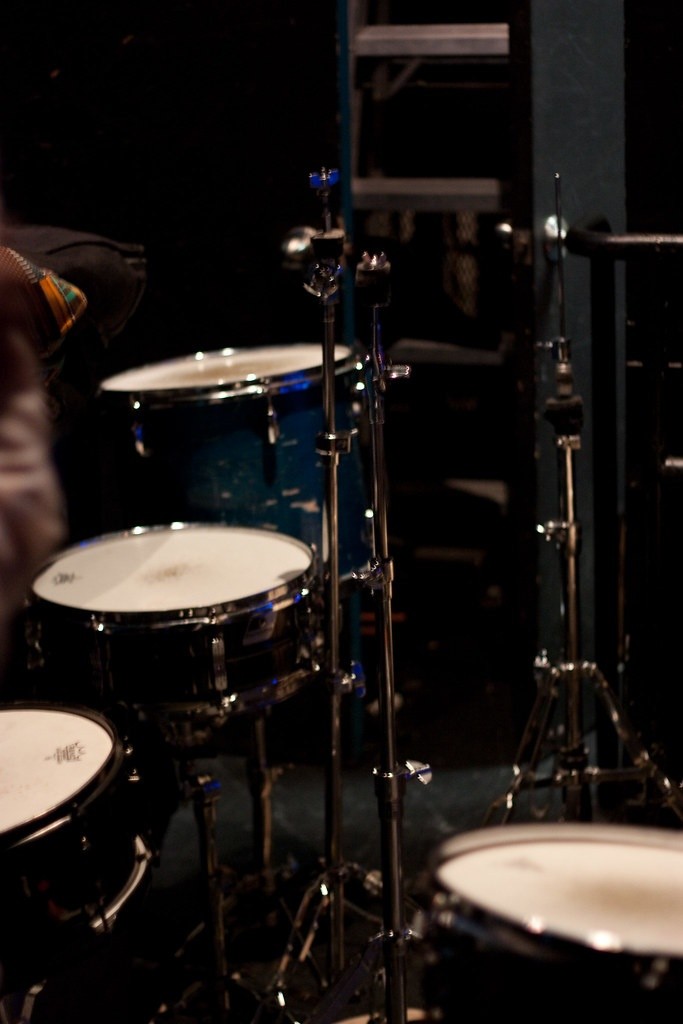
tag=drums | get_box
[0,696,151,979]
[416,818,682,1022]
[95,336,366,520]
[22,516,319,726]
